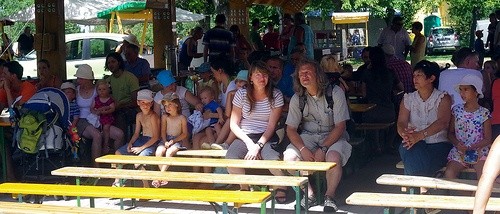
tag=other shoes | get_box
[160,180,168,187]
[6,193,70,204]
[112,178,125,187]
[152,180,160,188]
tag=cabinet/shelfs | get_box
[310,30,343,60]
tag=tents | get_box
[0,0,206,33]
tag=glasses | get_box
[470,47,476,54]
[422,62,433,69]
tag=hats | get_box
[235,70,248,81]
[454,74,484,98]
[280,13,294,23]
[476,30,483,36]
[161,91,179,101]
[122,34,140,47]
[195,62,211,73]
[382,44,395,55]
[60,82,77,91]
[74,64,94,79]
[156,70,176,87]
[137,89,153,101]
[251,20,260,26]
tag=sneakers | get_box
[295,194,318,210]
[211,142,230,150]
[200,143,212,150]
[324,195,338,212]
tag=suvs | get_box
[425,26,460,56]
[14,32,130,80]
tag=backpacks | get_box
[16,107,59,154]
[299,72,354,134]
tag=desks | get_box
[346,103,377,112]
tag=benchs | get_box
[345,160,500,214]
[0,119,396,214]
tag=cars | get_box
[472,20,500,50]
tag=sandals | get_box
[275,188,288,204]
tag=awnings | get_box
[332,12,369,24]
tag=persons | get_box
[187,55,288,204]
[74,64,124,168]
[473,134,500,214]
[115,34,141,61]
[106,52,140,146]
[109,89,161,202]
[152,94,190,188]
[320,11,427,157]
[90,79,116,155]
[0,26,80,199]
[123,45,152,85]
[153,70,205,139]
[397,60,452,214]
[438,8,500,142]
[445,73,492,214]
[177,11,316,100]
[283,60,352,212]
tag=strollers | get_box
[5,87,82,204]
